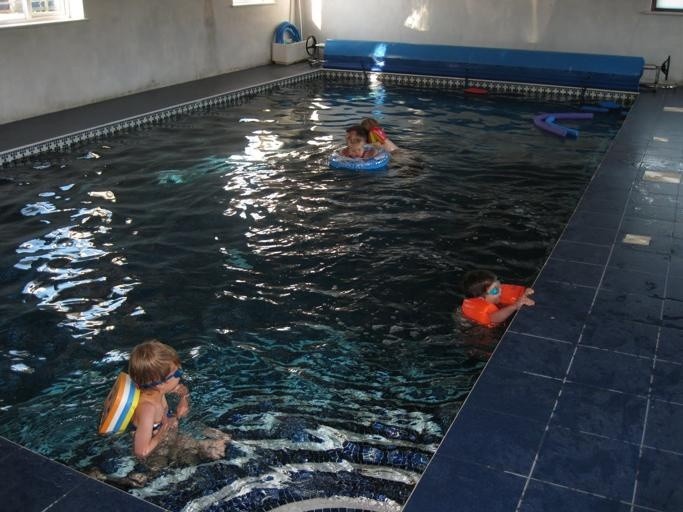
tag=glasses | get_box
[480,287,499,295]
[137,369,184,388]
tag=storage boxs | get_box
[271,37,314,67]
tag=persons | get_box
[337,117,385,161]
[460,269,536,329]
[128,339,228,477]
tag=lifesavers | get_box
[462,298,500,329]
[328,144,391,169]
[368,126,386,143]
[498,282,525,303]
